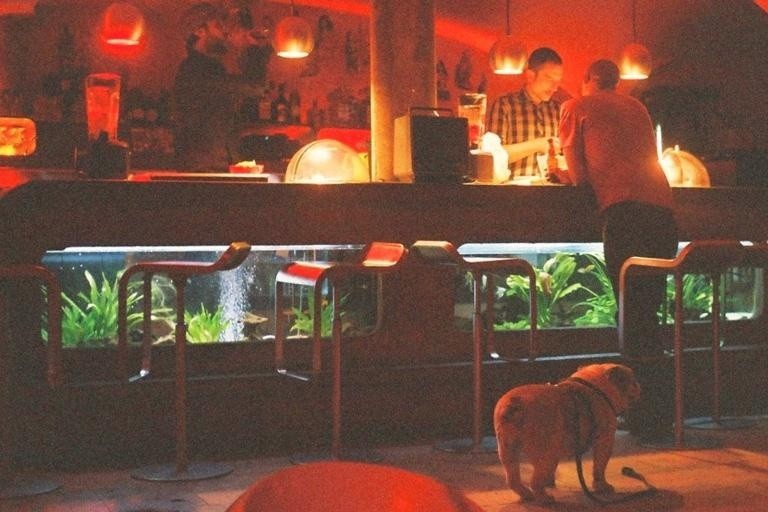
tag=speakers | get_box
[393,106,471,183]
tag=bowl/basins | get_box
[231,164,267,173]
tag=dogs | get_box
[492,360,642,507]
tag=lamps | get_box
[101,0,144,45]
[272,0,315,59]
[487,0,529,74]
[617,0,652,79]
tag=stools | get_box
[113,242,252,483]
[225,462,483,512]
[0,264,71,498]
[274,242,409,466]
[405,240,537,454]
[619,239,768,448]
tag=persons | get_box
[485,47,564,182]
[543,58,678,445]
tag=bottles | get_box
[546,139,560,183]
[129,90,161,125]
[245,81,303,125]
[309,87,370,130]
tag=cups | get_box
[84,73,123,141]
[457,93,488,150]
